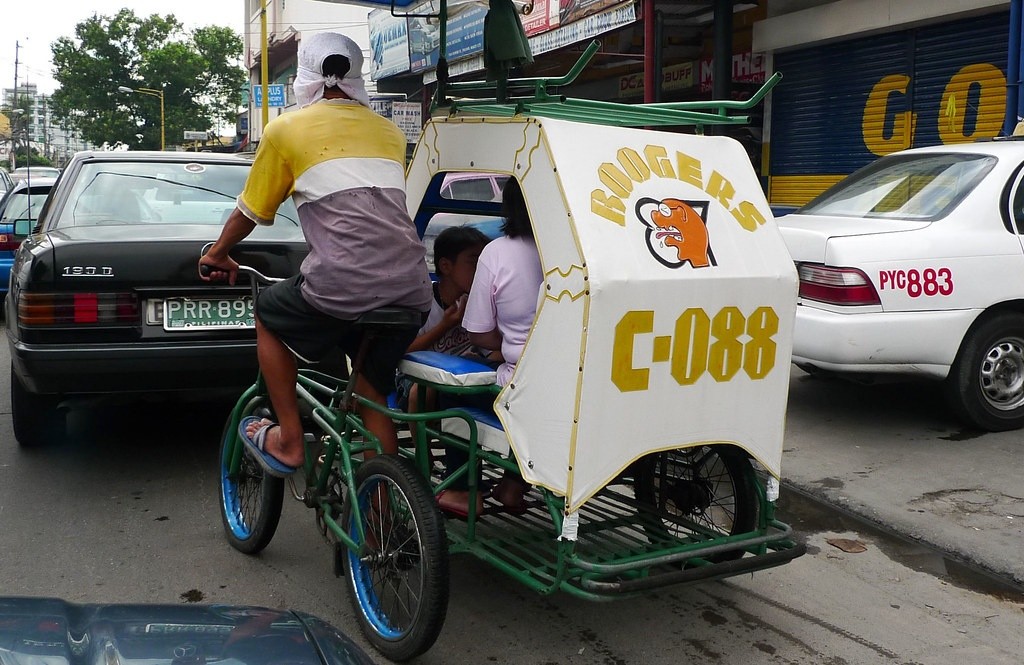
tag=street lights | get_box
[117,85,165,150]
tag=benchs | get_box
[398,351,512,458]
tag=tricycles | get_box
[214,1,810,665]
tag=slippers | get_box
[493,485,527,514]
[436,489,481,523]
[238,415,297,478]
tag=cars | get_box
[0,594,373,664]
[1,148,343,449]
[0,164,64,302]
[772,119,1024,431]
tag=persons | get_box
[395,175,544,518]
[198,32,433,555]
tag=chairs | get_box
[94,192,143,226]
